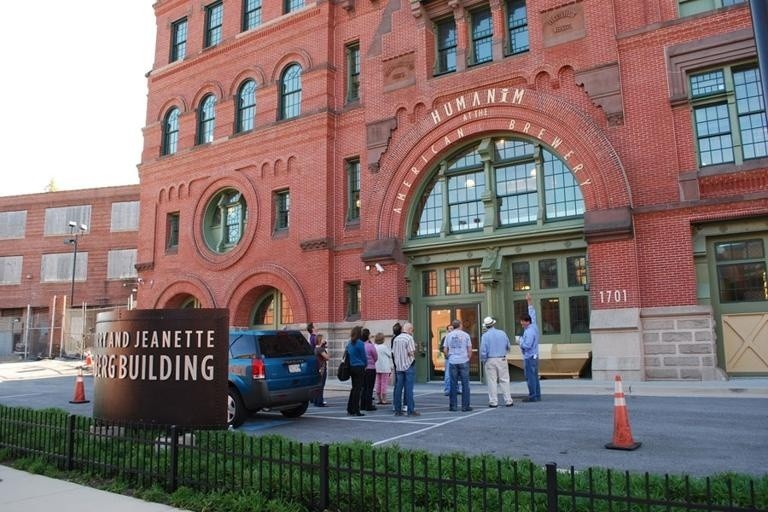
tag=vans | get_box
[14,341,55,360]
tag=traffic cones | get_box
[603,375,644,451]
[70,365,91,403]
[86,351,92,369]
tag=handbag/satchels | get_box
[338,363,350,380]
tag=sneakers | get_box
[522,398,536,402]
[396,406,420,416]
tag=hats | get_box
[482,317,496,326]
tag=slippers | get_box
[376,403,392,404]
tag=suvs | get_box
[226,327,324,428]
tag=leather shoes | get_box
[506,404,513,406]
[345,405,377,416]
[489,405,498,407]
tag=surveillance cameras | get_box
[137,278,143,282]
[375,263,384,272]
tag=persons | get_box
[443,320,473,412]
[517,292,541,402]
[307,322,421,418]
[479,316,514,407]
[439,325,463,396]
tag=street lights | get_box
[63,220,89,307]
